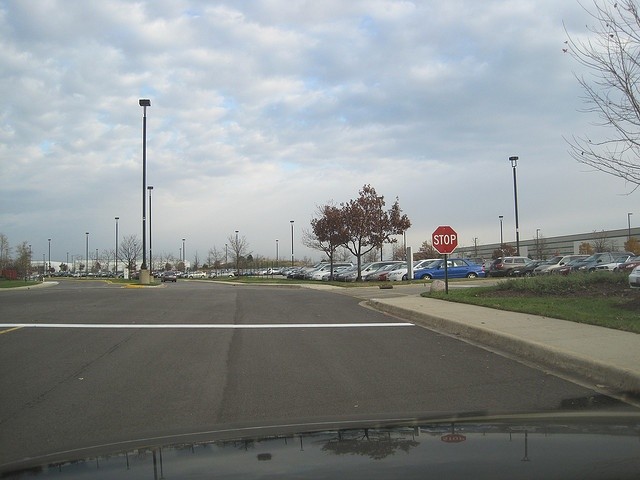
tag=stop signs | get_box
[431,225,458,254]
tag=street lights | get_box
[48,239,51,270]
[179,248,182,261]
[96,249,98,259]
[290,221,294,266]
[66,253,69,277]
[115,217,119,274]
[276,240,278,260]
[139,99,151,269]
[509,156,520,256]
[29,245,31,281]
[499,216,503,250]
[148,186,153,275]
[182,239,185,262]
[235,231,239,247]
[86,233,88,273]
[225,244,227,263]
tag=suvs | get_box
[174,271,181,278]
[187,270,207,280]
[489,256,532,277]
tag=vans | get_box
[306,262,329,280]
[533,255,590,276]
[313,263,353,281]
[355,261,407,281]
[272,267,280,275]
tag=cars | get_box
[322,266,347,282]
[161,271,177,282]
[209,271,234,278]
[619,256,640,272]
[628,265,640,288]
[366,265,393,281]
[388,259,457,281]
[507,260,550,277]
[551,258,587,276]
[241,271,252,276]
[594,255,636,272]
[414,258,486,280]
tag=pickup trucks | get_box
[569,253,615,273]
